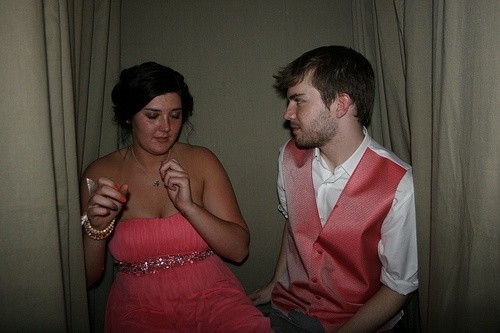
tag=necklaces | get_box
[129,146,171,187]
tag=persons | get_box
[246,46,417,333]
[80,61,274,333]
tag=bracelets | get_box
[81,176,116,240]
